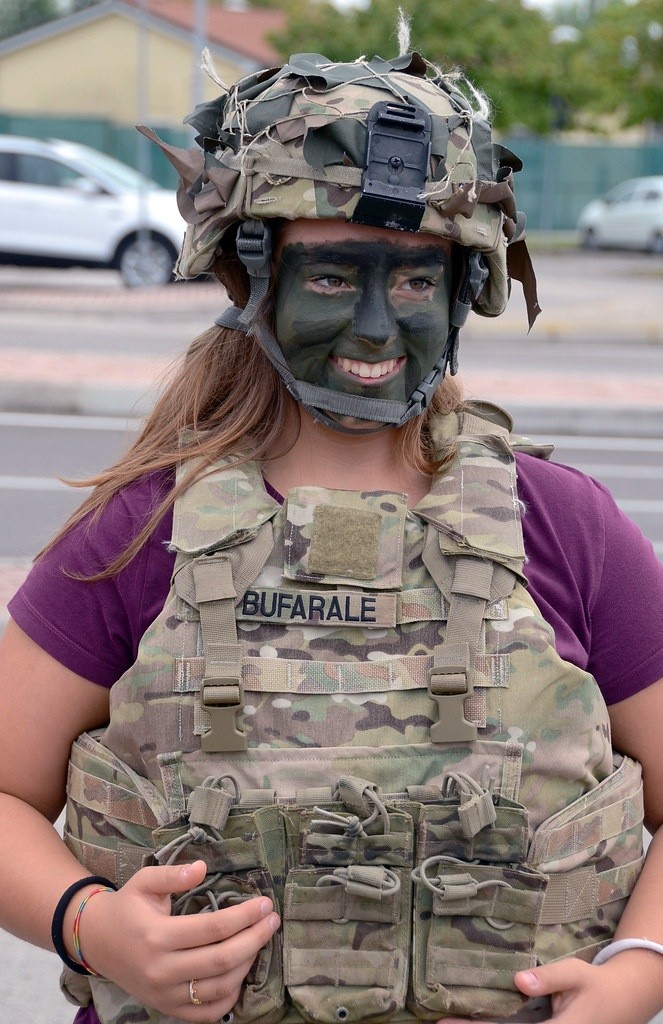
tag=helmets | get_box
[135,45,544,333]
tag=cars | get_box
[0,132,214,289]
[573,174,662,253]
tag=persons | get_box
[0,52,663,1024]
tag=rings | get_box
[189,980,202,1005]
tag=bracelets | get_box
[73,887,113,978]
[592,938,663,965]
[51,875,119,975]
